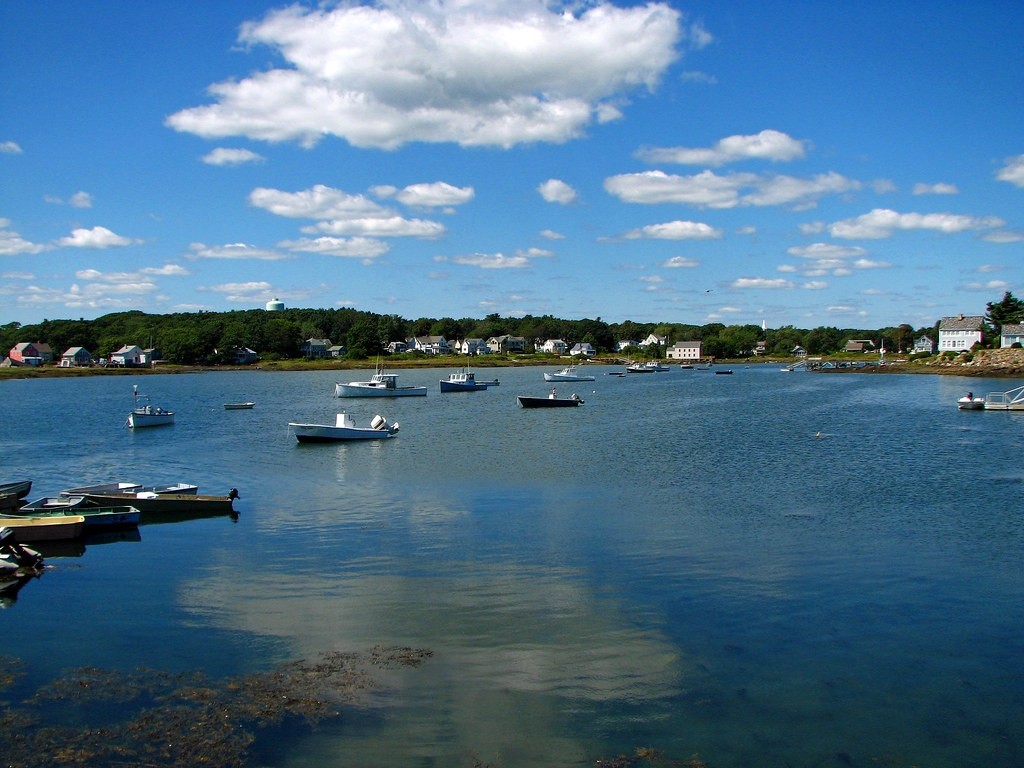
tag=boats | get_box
[223,402,256,410]
[439,358,487,393]
[0,515,86,538]
[61,482,143,494]
[697,367,710,370]
[0,504,141,527]
[0,480,33,502]
[59,482,197,497]
[957,392,984,410]
[128,385,175,428]
[542,358,595,382]
[609,372,623,375]
[60,488,241,509]
[475,378,501,387]
[517,388,585,408]
[334,352,428,397]
[715,369,734,374]
[0,527,45,591]
[288,409,400,443]
[625,351,671,374]
[681,365,695,369]
